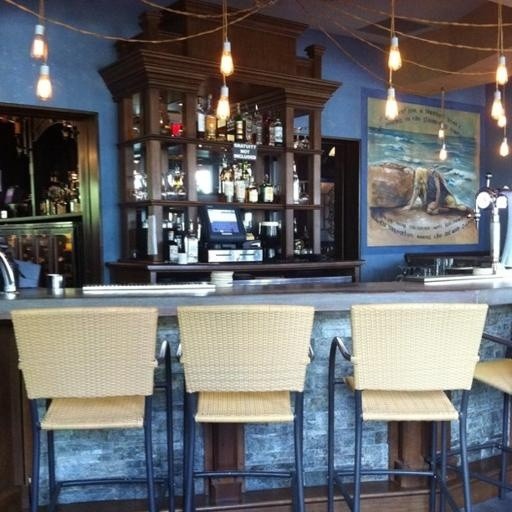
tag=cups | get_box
[48,290,65,298]
[43,272,64,289]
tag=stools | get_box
[452,356,512,504]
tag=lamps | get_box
[213,66,232,120]
[386,1,402,71]
[34,44,54,102]
[217,2,236,77]
[29,2,52,62]
[433,1,511,161]
[385,55,400,119]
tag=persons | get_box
[400,166,470,216]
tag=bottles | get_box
[130,217,148,260]
[196,94,283,147]
[277,218,282,256]
[170,163,185,199]
[158,213,199,263]
[220,150,275,204]
[291,217,310,249]
[246,219,258,241]
[293,161,301,204]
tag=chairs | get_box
[13,309,171,511]
[329,301,489,512]
[175,305,317,511]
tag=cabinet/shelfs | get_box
[109,60,341,264]
[0,219,83,287]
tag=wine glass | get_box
[293,126,306,149]
[298,178,312,205]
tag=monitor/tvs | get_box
[203,204,250,248]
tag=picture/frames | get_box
[358,88,489,253]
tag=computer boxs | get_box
[207,247,264,264]
